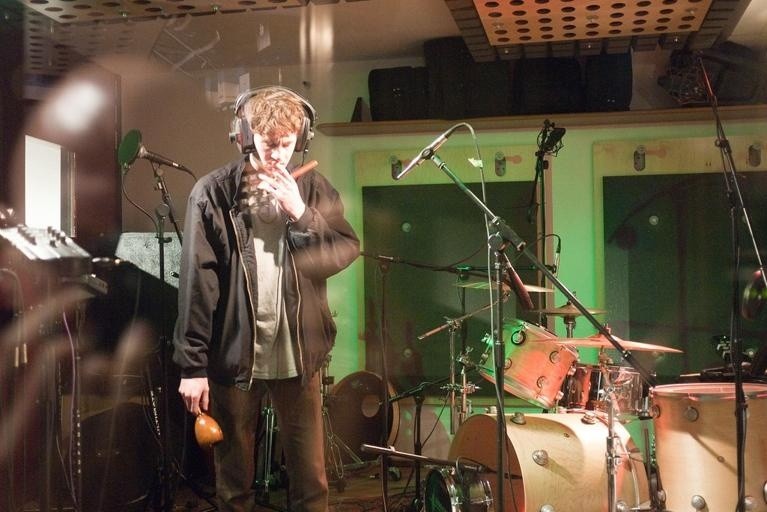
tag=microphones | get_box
[137,145,192,173]
[397,131,452,180]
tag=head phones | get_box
[227,85,317,154]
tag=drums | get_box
[566,360,641,416]
[446,413,653,512]
[649,381,766,512]
[422,468,494,512]
[475,318,580,404]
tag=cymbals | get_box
[529,295,607,317]
[528,324,684,355]
[454,278,557,294]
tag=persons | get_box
[171,87,361,512]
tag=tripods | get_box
[321,359,363,494]
[141,170,217,512]
